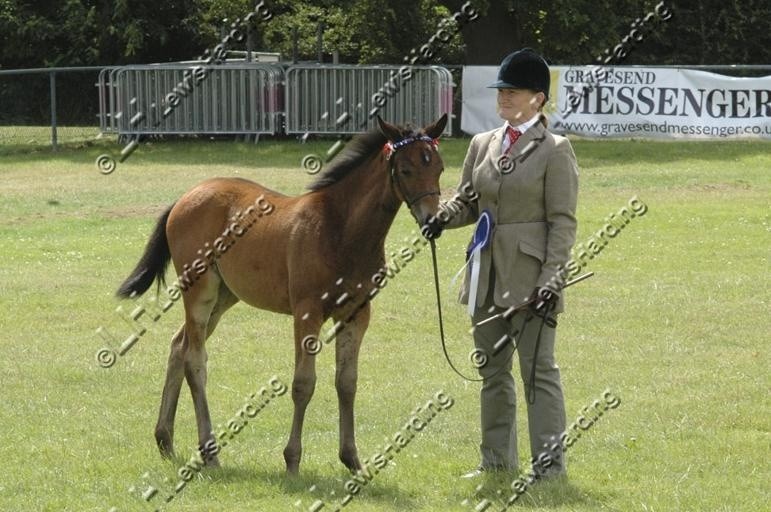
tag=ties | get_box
[507,127,521,150]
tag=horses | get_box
[115,108,453,478]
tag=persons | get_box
[435,48,581,487]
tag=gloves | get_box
[530,288,558,311]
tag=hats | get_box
[486,47,550,100]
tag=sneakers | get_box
[459,467,517,485]
